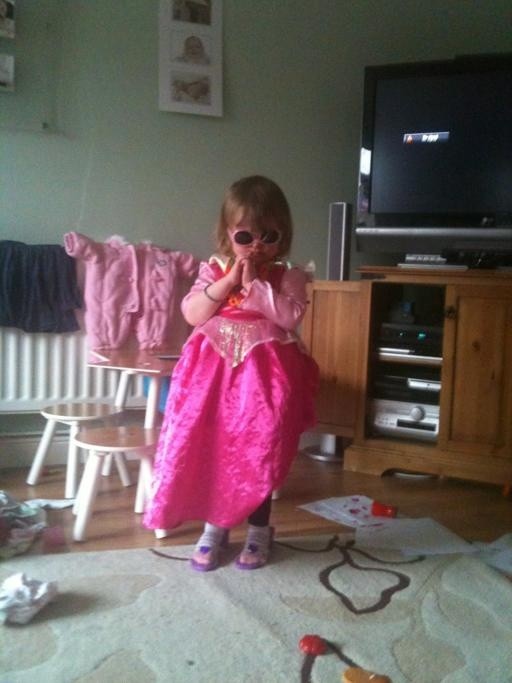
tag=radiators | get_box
[0,321,142,413]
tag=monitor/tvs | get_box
[355,52,512,251]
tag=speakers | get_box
[301,201,352,463]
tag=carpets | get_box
[2,517,512,682]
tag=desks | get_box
[91,344,184,513]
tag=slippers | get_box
[234,525,277,569]
[189,529,230,572]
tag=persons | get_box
[175,34,208,65]
[142,174,321,572]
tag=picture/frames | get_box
[157,0,224,117]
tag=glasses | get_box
[226,227,281,245]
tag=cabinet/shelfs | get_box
[298,262,511,484]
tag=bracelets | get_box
[202,284,225,304]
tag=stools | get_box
[26,405,132,499]
[72,423,171,541]
